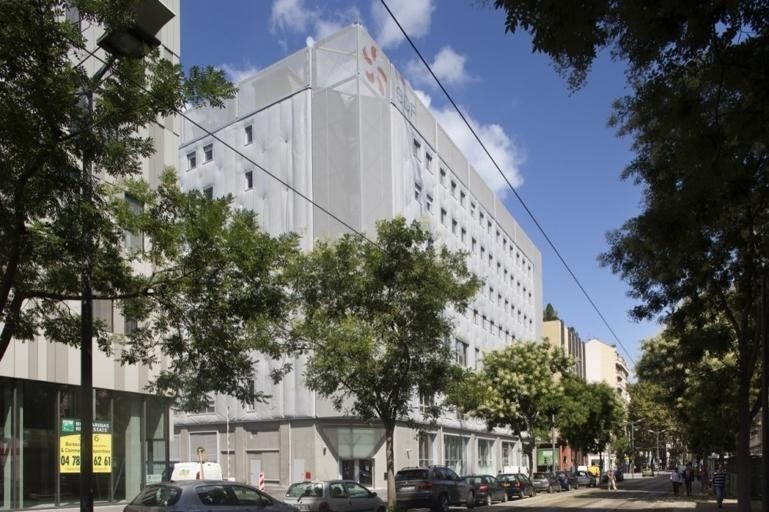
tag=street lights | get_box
[649,429,666,466]
[630,417,646,478]
[76,18,159,510]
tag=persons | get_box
[606,467,618,491]
[711,463,728,508]
[681,463,694,496]
[697,466,709,493]
[670,467,683,497]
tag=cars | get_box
[123,480,299,512]
[395,466,596,510]
[603,470,623,482]
[285,479,386,510]
[641,467,654,476]
